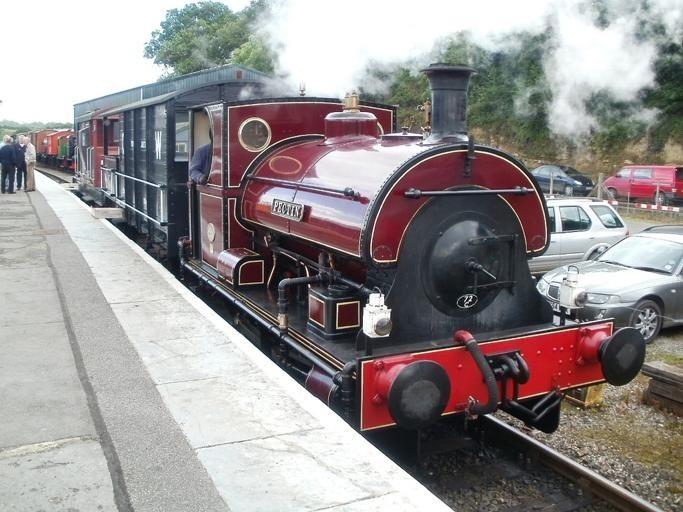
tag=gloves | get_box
[197,175,209,185]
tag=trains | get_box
[30,127,76,171]
[72,61,645,464]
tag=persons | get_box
[184,128,212,187]
[0,134,36,194]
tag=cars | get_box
[526,198,629,275]
[535,225,683,344]
[530,165,596,197]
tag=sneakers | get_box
[17,187,35,192]
[2,190,16,194]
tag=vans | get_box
[604,164,683,205]
